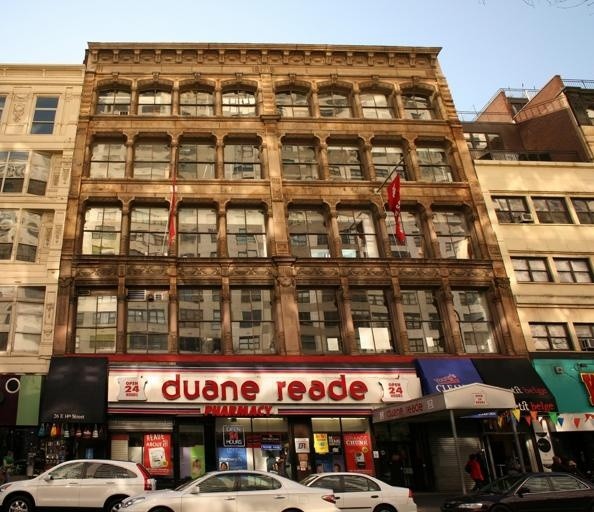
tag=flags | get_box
[386,175,407,240]
[167,172,179,246]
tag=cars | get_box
[0,459,156,512]
[111,469,340,512]
[441,471,594,512]
[298,472,419,512]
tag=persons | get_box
[505,456,518,475]
[473,452,490,485]
[276,451,291,481]
[333,464,341,472]
[466,454,484,491]
[542,456,566,472]
[3,449,15,483]
[562,455,576,474]
[192,459,203,476]
[388,450,405,485]
[221,462,227,470]
